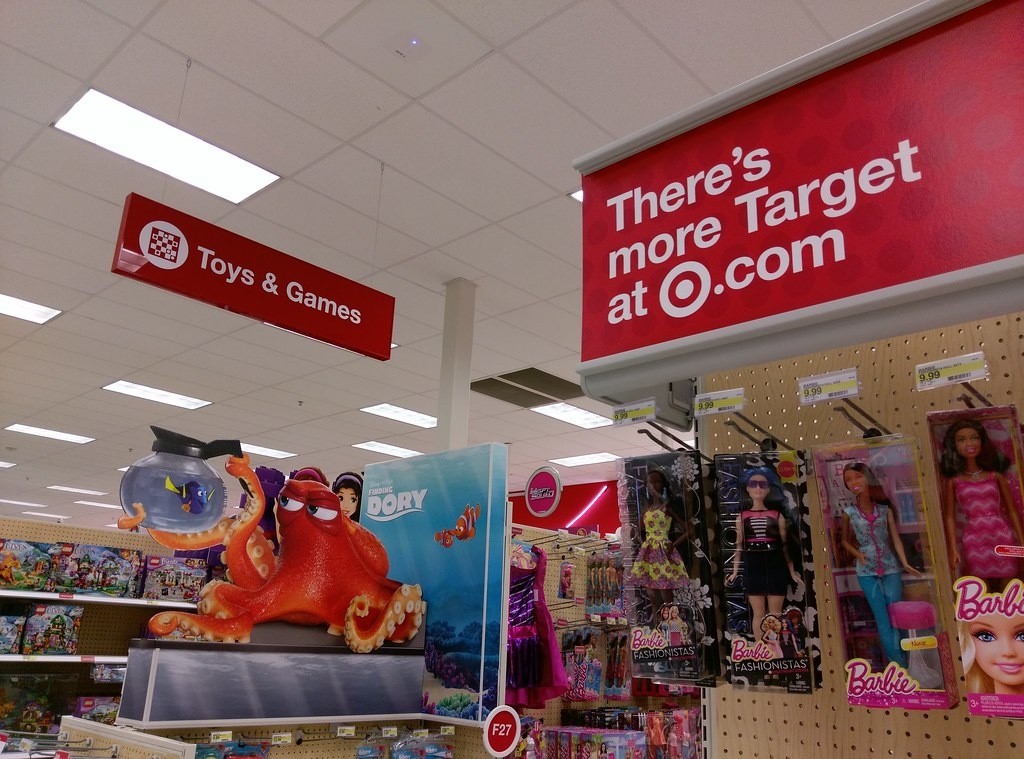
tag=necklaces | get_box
[856,501,885,575]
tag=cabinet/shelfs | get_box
[0,518,175,759]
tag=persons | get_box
[729,467,804,648]
[627,470,695,651]
[935,414,1022,592]
[840,460,923,673]
[565,636,598,692]
[625,709,702,759]
[589,558,623,607]
[607,636,629,696]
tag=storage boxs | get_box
[0,674,80,752]
[0,602,85,655]
[0,538,207,604]
[68,696,120,727]
[546,726,647,759]
